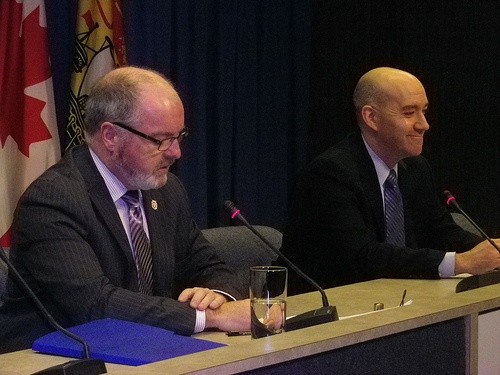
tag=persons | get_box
[302,66,500,292]
[1,66,283,358]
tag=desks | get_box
[0,270,500,375]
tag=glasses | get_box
[112,122,193,151]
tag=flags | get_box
[64,0,127,151]
[0,0,63,290]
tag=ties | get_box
[384,168,405,247]
[122,190,153,295]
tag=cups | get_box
[249,266,287,339]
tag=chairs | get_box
[199,225,284,298]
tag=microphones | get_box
[0,246,106,375]
[222,199,339,332]
[441,190,500,293]
